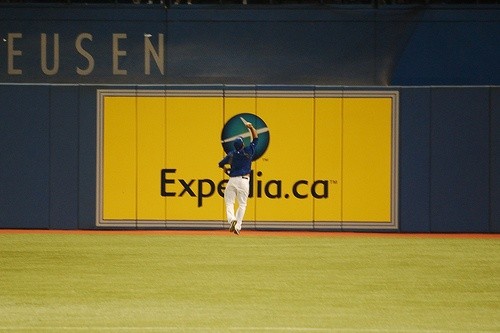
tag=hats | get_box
[234,137,243,147]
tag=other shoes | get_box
[228,219,237,232]
[234,228,240,236]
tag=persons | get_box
[218,121,259,236]
